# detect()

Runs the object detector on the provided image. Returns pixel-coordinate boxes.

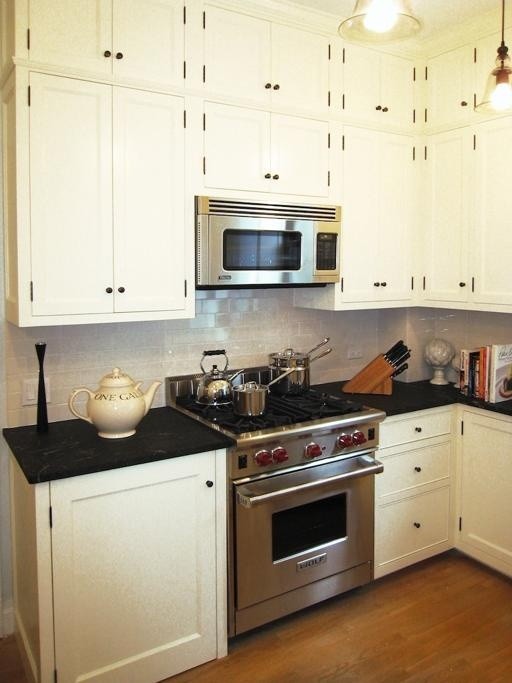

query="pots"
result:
[231,366,295,417]
[269,336,333,394]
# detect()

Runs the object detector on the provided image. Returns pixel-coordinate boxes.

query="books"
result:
[459,344,512,404]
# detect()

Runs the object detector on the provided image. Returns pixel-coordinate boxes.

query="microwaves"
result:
[195,195,341,290]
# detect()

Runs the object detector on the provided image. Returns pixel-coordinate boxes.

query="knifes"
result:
[384,340,411,378]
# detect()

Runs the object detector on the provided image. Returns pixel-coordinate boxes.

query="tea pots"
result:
[194,348,246,405]
[69,366,162,438]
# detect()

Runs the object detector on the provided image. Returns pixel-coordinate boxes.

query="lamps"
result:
[479,0,512,110]
[332,0,425,45]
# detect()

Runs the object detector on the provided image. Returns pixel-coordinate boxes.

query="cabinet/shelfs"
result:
[417,117,510,316]
[293,129,416,311]
[0,0,188,85]
[418,24,512,134]
[11,450,226,683]
[458,404,512,579]
[0,70,198,328]
[375,400,458,580]
[192,1,334,112]
[195,100,337,207]
[328,37,420,135]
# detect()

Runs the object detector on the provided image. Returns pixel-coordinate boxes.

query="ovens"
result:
[227,446,387,637]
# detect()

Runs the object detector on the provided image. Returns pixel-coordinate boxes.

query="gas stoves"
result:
[176,387,387,479]
